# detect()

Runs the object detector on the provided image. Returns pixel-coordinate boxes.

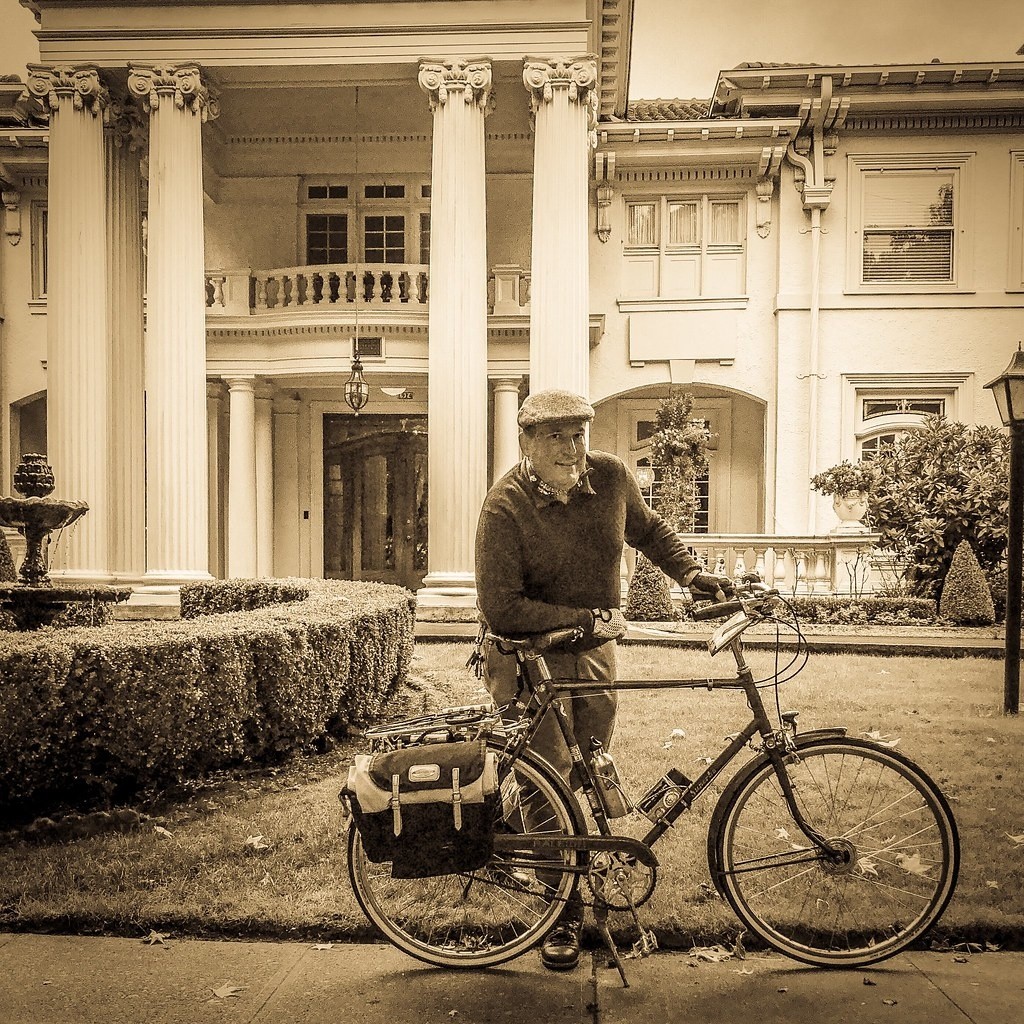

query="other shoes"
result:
[487,848,532,887]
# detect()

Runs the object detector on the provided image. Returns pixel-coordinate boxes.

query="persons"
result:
[474,387,737,970]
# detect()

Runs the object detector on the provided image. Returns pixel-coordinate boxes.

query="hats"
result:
[517,387,596,428]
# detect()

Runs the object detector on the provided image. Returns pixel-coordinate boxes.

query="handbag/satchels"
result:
[339,740,518,880]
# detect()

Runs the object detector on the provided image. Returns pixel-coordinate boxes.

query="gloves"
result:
[681,569,738,605]
[593,607,628,639]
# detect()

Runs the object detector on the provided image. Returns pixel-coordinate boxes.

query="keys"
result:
[465,649,484,680]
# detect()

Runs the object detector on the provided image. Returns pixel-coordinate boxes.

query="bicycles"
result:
[349,576,961,1023]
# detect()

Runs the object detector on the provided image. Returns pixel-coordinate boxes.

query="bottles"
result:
[588,735,633,818]
[636,768,691,824]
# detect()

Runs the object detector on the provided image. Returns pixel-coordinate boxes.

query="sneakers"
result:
[543,888,585,967]
